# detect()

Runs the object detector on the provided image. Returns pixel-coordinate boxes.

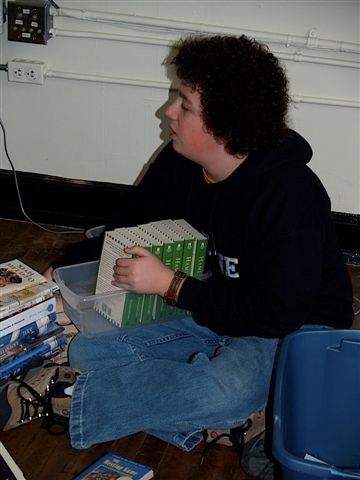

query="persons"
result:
[1,34,354,478]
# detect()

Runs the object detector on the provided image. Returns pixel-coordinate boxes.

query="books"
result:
[0,258,67,379]
[93,219,209,331]
[73,453,153,480]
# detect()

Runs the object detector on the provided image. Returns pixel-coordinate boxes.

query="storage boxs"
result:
[53,261,214,338]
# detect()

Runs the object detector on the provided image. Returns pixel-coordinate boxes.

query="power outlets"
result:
[8,59,46,84]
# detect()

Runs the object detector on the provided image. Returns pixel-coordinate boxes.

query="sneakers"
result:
[203,406,274,478]
[0,356,80,432]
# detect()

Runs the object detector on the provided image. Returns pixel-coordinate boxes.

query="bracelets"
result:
[163,270,187,307]
